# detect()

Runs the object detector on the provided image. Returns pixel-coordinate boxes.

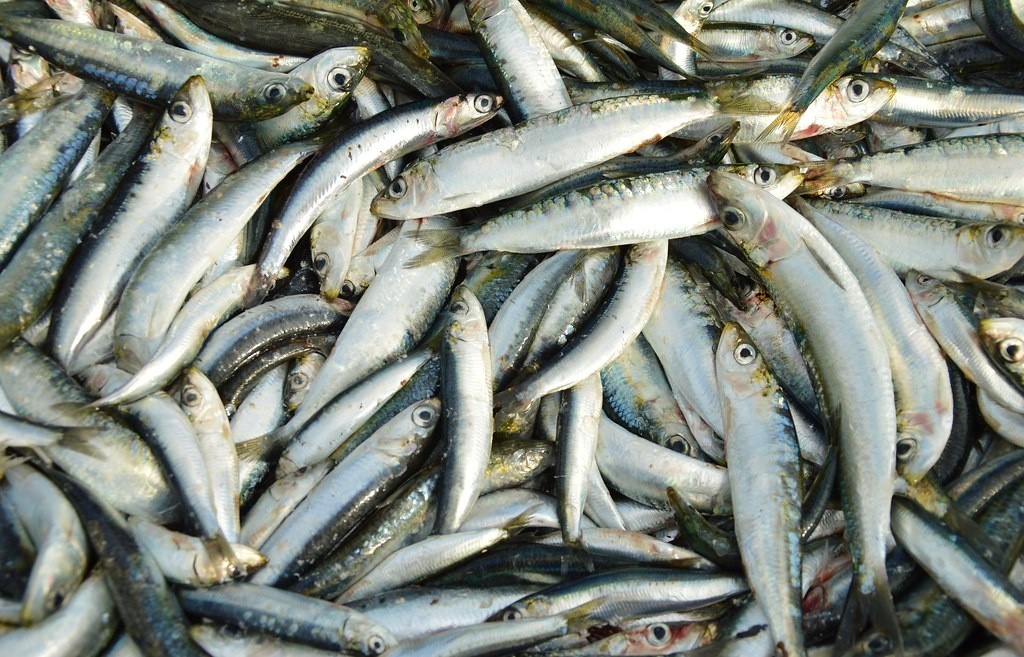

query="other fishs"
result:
[0,0,1024,657]
